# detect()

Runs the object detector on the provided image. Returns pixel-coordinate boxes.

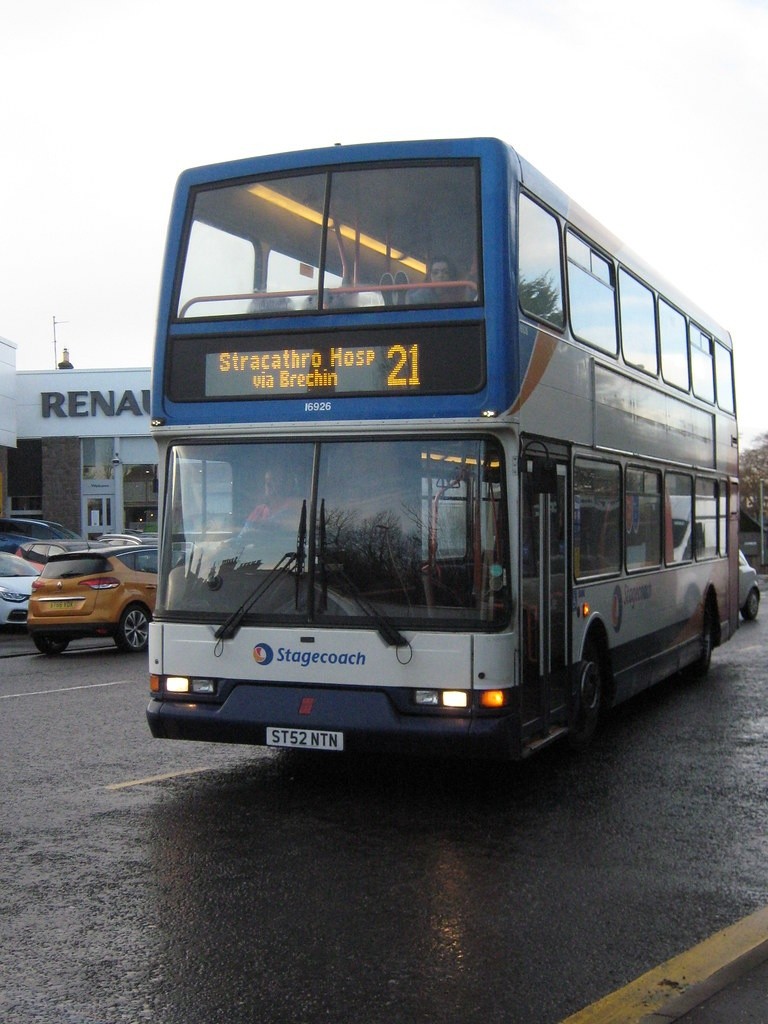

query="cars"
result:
[27,544,188,655]
[0,518,84,556]
[14,539,113,575]
[738,549,761,621]
[0,551,43,630]
[96,529,160,548]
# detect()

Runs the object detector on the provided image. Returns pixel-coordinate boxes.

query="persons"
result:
[216,462,307,569]
[379,255,466,305]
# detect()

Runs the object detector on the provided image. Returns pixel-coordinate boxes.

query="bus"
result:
[149,136,742,759]
